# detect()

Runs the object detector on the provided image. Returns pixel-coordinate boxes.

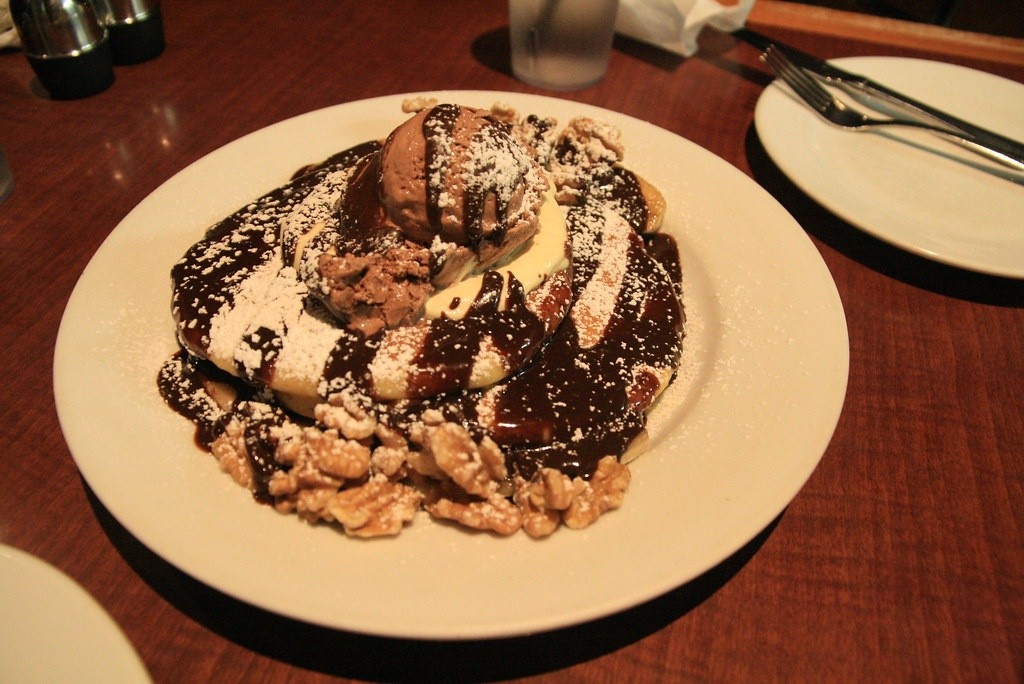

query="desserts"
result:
[154,95,687,540]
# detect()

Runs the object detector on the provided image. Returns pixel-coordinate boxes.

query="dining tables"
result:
[5,0,1021,684]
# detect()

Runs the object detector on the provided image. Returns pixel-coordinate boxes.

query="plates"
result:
[51,91,850,639]
[0,542,152,684]
[752,56,1024,279]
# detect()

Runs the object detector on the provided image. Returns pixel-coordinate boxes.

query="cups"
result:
[509,0,620,90]
[8,1,116,100]
[89,0,166,68]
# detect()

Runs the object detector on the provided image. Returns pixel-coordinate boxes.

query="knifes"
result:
[731,26,1023,160]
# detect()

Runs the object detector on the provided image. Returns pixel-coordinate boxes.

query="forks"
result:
[760,44,1024,169]
